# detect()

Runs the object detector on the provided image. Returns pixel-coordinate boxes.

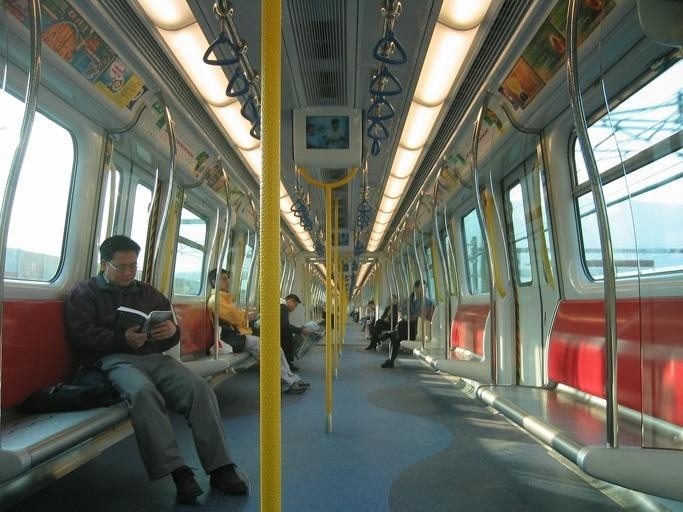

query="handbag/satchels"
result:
[416,317,430,342]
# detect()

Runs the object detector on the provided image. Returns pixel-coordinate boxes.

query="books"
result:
[114,306,171,343]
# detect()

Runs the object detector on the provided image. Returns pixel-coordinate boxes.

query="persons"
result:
[207,269,313,393]
[351,280,432,368]
[279,294,309,370]
[67,235,247,501]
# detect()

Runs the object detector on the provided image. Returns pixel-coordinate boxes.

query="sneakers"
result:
[283,364,312,397]
[169,466,202,501]
[364,333,396,370]
[210,463,247,494]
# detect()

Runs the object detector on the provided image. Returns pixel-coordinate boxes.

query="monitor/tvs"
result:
[343,262,351,297]
[293,105,363,168]
[331,229,355,250]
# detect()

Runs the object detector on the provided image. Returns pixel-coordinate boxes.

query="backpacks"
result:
[28,361,119,412]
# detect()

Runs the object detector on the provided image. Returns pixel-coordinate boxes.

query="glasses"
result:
[104,259,139,273]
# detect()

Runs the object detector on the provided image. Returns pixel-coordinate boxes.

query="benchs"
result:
[474,296,682,501]
[399,295,495,387]
[0,294,263,512]
